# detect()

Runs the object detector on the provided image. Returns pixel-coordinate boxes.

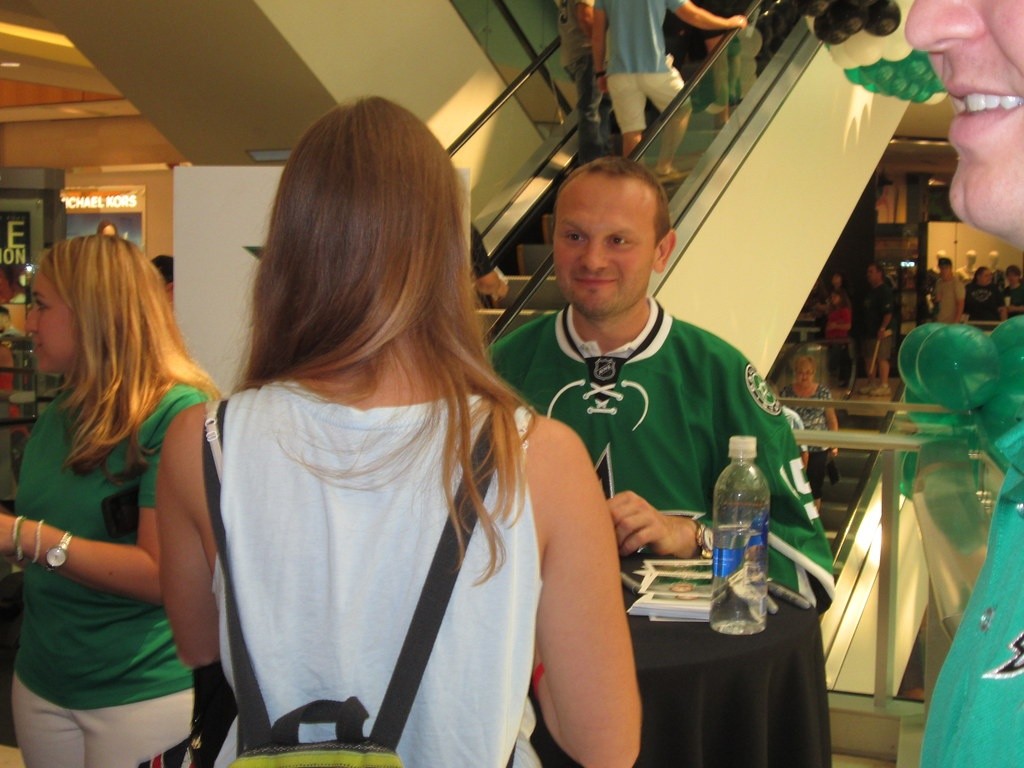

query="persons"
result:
[551,0,756,191]
[0,234,228,768]
[763,255,1024,513]
[157,95,642,768]
[905,0,1024,768]
[485,154,835,618]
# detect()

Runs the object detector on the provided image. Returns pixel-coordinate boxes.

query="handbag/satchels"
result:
[826,459,840,486]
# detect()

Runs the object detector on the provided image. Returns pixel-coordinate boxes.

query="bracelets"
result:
[12,516,27,563]
[32,520,45,564]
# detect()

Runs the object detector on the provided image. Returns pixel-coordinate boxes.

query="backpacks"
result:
[166,393,511,768]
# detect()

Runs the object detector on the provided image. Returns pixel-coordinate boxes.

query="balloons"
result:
[737,0,804,95]
[796,0,951,106]
[894,313,1024,551]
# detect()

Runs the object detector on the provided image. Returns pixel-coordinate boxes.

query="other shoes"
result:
[653,164,680,184]
[858,383,891,396]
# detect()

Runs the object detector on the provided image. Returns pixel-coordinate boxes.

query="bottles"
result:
[709,434,768,635]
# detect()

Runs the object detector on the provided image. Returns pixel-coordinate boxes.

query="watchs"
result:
[45,530,72,575]
[695,521,714,559]
